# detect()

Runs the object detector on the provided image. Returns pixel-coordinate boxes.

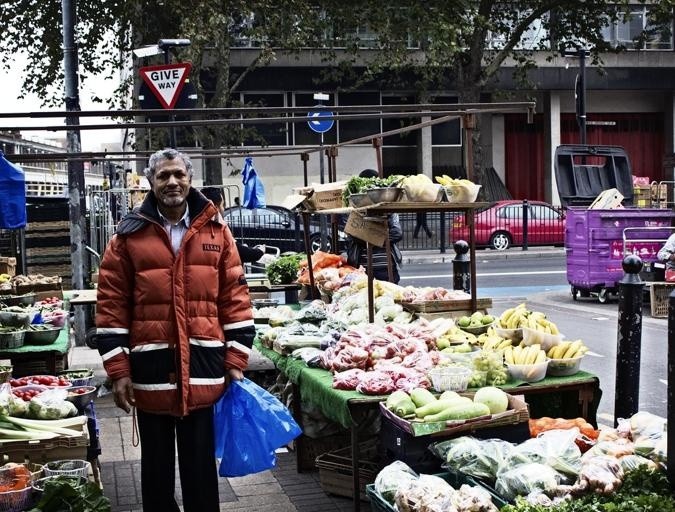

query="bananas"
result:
[441,303,590,378]
[435,173,476,201]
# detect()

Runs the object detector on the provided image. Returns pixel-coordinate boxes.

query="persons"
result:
[413,212,435,239]
[657,232,675,272]
[96,149,258,510]
[337,170,404,287]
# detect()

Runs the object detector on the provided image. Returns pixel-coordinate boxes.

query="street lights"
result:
[558,40,591,166]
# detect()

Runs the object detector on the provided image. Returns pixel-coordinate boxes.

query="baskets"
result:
[650,284,674,317]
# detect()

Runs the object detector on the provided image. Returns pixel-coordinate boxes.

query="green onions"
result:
[0,413,87,443]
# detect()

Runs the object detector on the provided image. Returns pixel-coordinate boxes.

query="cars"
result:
[222,206,348,254]
[449,198,567,250]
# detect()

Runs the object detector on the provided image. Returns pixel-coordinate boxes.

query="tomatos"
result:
[9,375,89,401]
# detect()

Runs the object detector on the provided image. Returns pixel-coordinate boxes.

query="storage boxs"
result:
[380,384,532,472]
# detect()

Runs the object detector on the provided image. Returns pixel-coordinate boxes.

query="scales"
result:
[245,273,271,290]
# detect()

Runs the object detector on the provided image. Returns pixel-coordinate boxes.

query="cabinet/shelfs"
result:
[290,196,492,332]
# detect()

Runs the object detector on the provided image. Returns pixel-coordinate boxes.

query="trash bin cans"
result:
[554,145,675,303]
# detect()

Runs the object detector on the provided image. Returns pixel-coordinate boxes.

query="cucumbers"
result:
[386,388,507,423]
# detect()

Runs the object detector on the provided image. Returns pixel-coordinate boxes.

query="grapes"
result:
[468,352,512,387]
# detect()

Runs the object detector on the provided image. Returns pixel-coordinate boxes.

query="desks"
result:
[251,337,602,510]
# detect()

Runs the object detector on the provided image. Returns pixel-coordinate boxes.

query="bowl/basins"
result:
[0,457,91,510]
[10,369,98,410]
[0,290,61,348]
[345,184,482,209]
[429,312,587,391]
